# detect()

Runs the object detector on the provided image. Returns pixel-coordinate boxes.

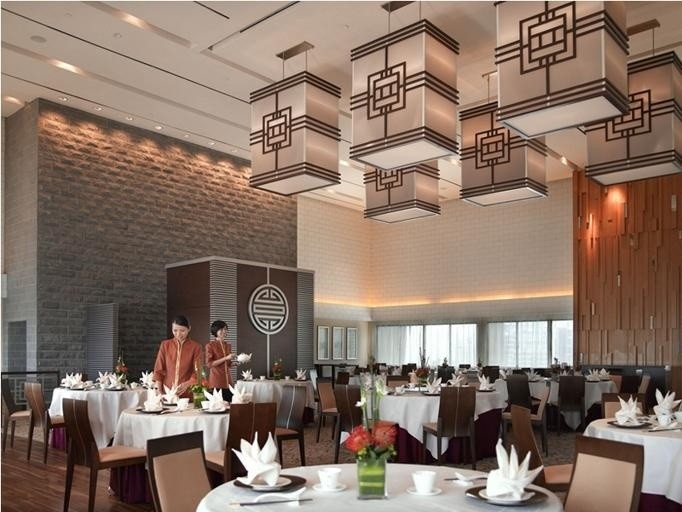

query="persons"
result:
[153,315,203,403]
[205,320,238,400]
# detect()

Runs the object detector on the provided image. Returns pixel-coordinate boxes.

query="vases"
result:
[419,378,428,389]
[356,459,387,499]
[275,373,282,380]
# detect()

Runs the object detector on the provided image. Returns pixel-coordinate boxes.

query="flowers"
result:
[416,347,436,378]
[345,355,398,460]
[273,358,283,373]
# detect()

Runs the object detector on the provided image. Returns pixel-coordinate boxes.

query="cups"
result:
[412,471,437,493]
[395,386,406,394]
[86,381,92,385]
[130,382,140,390]
[318,468,342,488]
[179,398,189,410]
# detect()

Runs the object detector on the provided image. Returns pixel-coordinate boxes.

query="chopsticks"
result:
[230,499,314,507]
[444,477,488,481]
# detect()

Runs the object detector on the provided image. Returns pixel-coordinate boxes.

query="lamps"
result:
[582,23,677,187]
[347,1,459,172]
[458,73,549,208]
[247,46,341,197]
[361,160,441,224]
[495,2,633,140]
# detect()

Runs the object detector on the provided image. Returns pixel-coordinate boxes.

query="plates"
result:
[141,409,163,414]
[313,484,346,492]
[202,408,230,414]
[234,475,306,493]
[607,420,652,428]
[422,392,440,396]
[407,487,442,496]
[477,388,496,392]
[465,486,550,506]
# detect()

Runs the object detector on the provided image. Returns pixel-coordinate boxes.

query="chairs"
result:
[510,404,574,492]
[333,385,364,463]
[501,380,552,457]
[512,364,571,379]
[563,432,644,512]
[277,385,307,467]
[205,401,277,470]
[320,361,417,393]
[601,391,647,422]
[102,396,103,398]
[60,397,147,492]
[145,430,213,492]
[316,376,341,442]
[22,380,66,463]
[506,374,541,410]
[554,376,586,434]
[2,377,37,454]
[422,386,477,468]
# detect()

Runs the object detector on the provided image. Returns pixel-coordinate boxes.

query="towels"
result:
[448,373,463,387]
[560,370,569,376]
[140,371,153,384]
[528,371,537,381]
[654,388,681,427]
[96,371,110,385]
[615,395,642,425]
[65,372,73,386]
[487,437,544,500]
[229,384,251,403]
[424,377,442,394]
[477,374,495,390]
[202,388,226,411]
[587,368,600,382]
[295,369,307,380]
[241,368,252,380]
[109,373,123,389]
[164,383,178,404]
[144,387,162,411]
[600,368,609,379]
[70,373,83,387]
[231,431,281,487]
